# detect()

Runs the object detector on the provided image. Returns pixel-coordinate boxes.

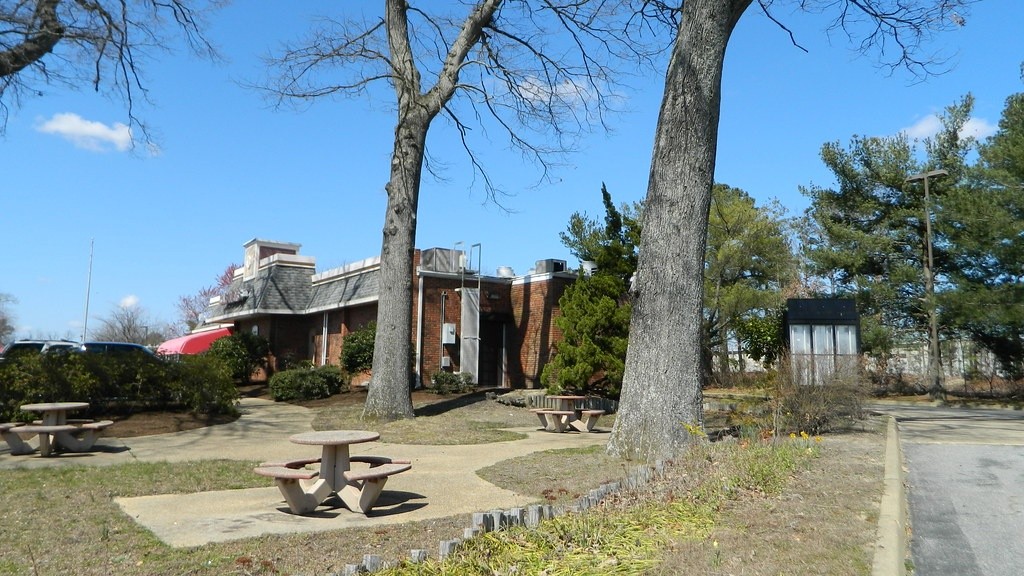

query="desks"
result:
[289,430,380,514]
[545,394,586,433]
[20,401,90,454]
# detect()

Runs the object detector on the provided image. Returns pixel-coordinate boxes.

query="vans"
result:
[0,339,81,383]
[40,341,187,407]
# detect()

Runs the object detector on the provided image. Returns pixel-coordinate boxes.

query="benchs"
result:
[529,407,605,433]
[253,453,412,514]
[0,418,114,456]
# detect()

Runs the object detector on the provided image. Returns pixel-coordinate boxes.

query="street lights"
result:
[906,167,952,398]
